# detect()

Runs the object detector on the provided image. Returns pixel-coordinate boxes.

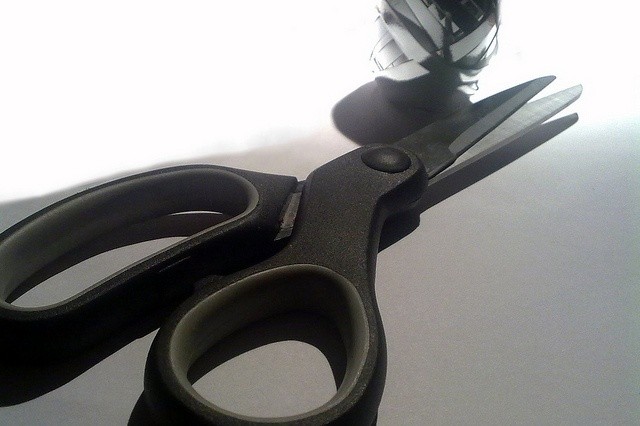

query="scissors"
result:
[1,75,582,426]
